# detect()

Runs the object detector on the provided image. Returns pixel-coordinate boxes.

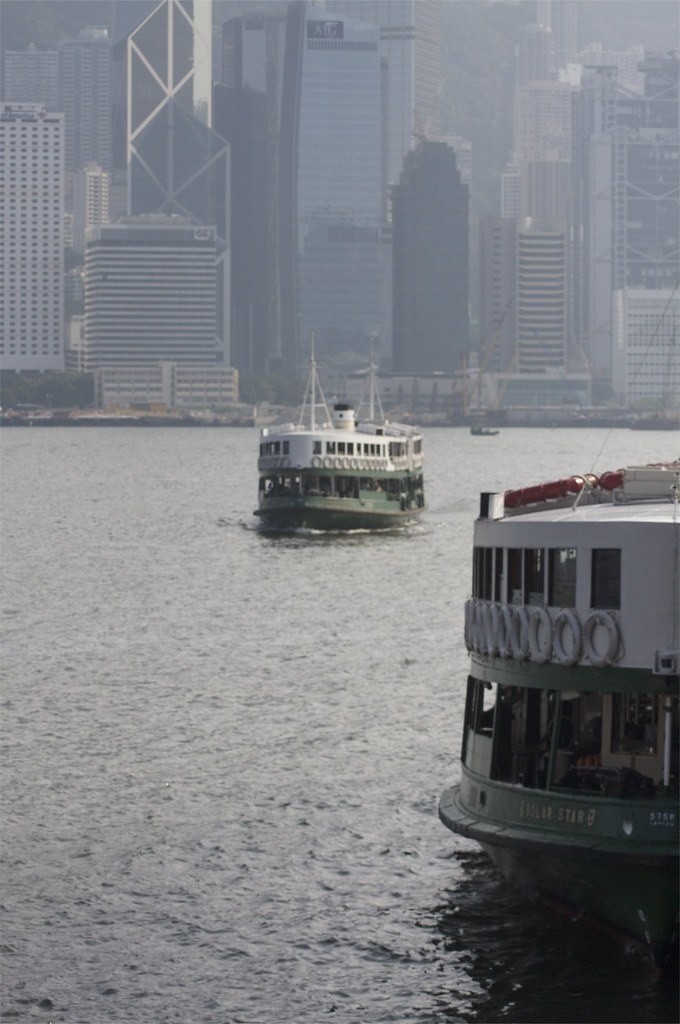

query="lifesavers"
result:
[582,612,620,668]
[257,456,291,469]
[343,457,389,471]
[528,607,553,665]
[553,608,582,668]
[333,457,342,469]
[311,456,322,468]
[510,606,529,661]
[323,457,333,469]
[463,599,509,657]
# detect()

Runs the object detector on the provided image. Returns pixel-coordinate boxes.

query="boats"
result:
[252,330,429,535]
[434,453,680,964]
